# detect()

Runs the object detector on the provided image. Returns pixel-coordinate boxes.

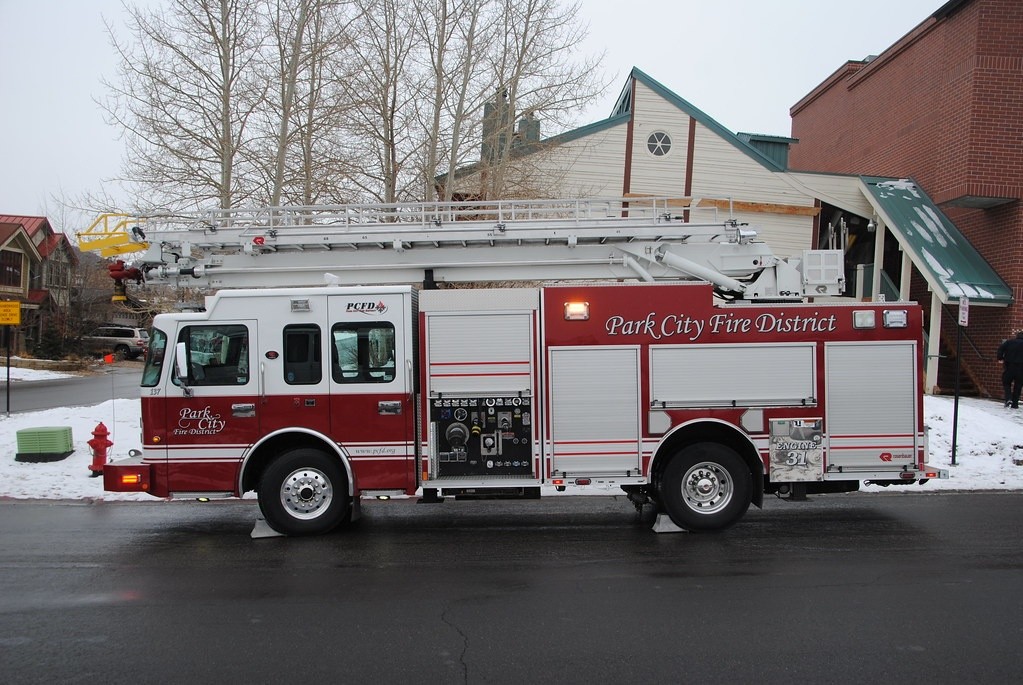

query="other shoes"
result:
[1004,398,1013,407]
[1011,406,1019,408]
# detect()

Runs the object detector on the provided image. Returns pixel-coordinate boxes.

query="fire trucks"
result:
[74,193,957,541]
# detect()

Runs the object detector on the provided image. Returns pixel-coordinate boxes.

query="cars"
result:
[143,339,166,365]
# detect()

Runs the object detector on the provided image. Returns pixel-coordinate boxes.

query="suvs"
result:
[78,324,151,362]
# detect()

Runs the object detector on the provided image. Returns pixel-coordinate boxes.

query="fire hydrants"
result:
[86,421,115,477]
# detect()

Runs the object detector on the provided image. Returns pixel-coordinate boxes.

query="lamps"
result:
[868,218,878,232]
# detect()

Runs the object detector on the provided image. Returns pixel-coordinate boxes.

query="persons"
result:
[997,331,1023,409]
